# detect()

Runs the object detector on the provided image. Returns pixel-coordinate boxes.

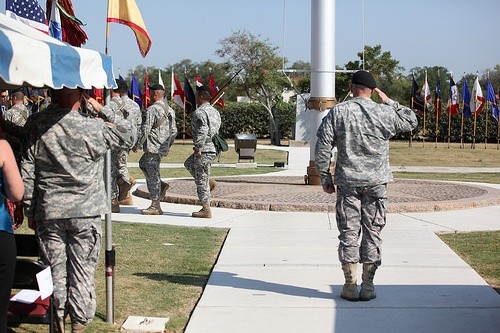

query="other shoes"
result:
[71,323,83,333]
[52,318,65,333]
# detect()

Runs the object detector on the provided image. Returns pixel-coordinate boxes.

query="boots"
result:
[140,200,162,215]
[192,201,212,218]
[130,177,136,188]
[160,181,169,198]
[359,264,376,301]
[118,190,132,205]
[341,262,360,301]
[208,179,217,191]
[111,199,120,213]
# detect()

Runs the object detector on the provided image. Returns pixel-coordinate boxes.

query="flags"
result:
[5,0,224,113]
[410,71,500,121]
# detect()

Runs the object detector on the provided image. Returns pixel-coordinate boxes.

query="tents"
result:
[0,13,118,89]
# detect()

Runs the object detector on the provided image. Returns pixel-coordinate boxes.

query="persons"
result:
[0,84,220,333]
[316,71,419,301]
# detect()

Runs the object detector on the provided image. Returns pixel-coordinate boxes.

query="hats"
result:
[150,85,164,90]
[352,70,377,90]
[199,86,213,95]
[113,78,121,87]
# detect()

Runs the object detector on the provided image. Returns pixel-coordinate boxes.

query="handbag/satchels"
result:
[212,133,228,155]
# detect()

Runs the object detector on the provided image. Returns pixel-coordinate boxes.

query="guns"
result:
[209,69,242,107]
